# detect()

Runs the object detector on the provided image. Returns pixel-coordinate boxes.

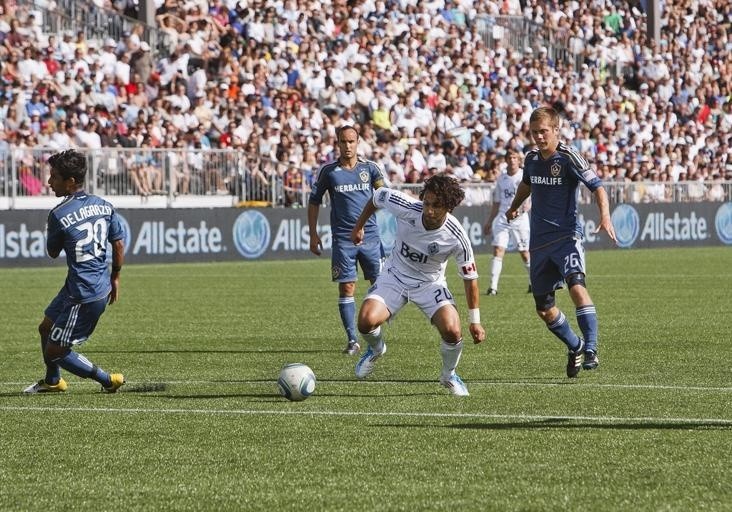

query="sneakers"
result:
[486,288,496,294]
[23,377,68,393]
[343,339,361,355]
[583,348,599,370]
[567,337,586,377]
[355,340,387,379]
[440,374,469,395]
[100,373,124,393]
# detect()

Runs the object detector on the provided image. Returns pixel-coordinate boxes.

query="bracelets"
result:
[468,308,481,323]
[112,264,121,271]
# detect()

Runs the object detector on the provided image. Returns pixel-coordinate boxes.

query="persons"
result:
[351,175,486,395]
[308,126,389,357]
[484,149,533,295]
[24,149,126,393]
[1,1,732,210]
[505,107,618,378]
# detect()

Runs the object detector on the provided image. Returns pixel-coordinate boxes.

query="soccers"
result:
[278,363,316,401]
